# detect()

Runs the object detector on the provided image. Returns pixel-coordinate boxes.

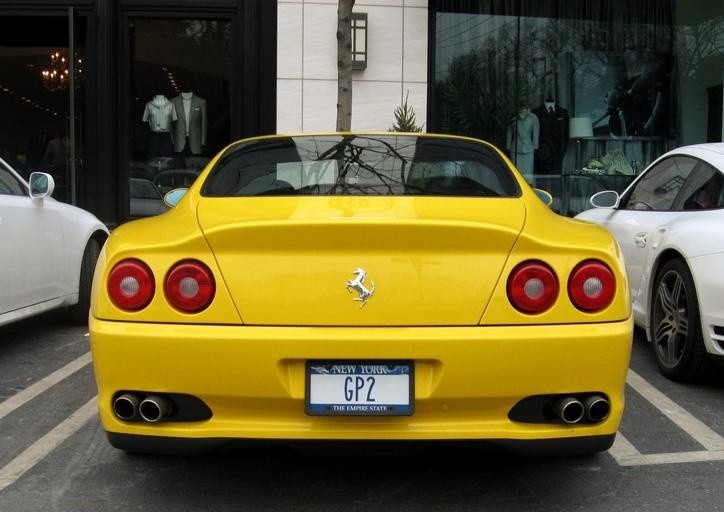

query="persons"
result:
[141,94,178,155]
[504,103,541,178]
[167,91,209,158]
[530,97,569,176]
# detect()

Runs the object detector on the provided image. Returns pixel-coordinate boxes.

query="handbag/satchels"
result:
[608,150,635,176]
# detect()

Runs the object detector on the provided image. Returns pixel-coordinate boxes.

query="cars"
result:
[571,140,724,383]
[0,154,113,333]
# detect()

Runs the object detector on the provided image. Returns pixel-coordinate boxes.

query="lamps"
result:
[40,50,87,93]
[569,117,593,175]
[351,12,368,70]
